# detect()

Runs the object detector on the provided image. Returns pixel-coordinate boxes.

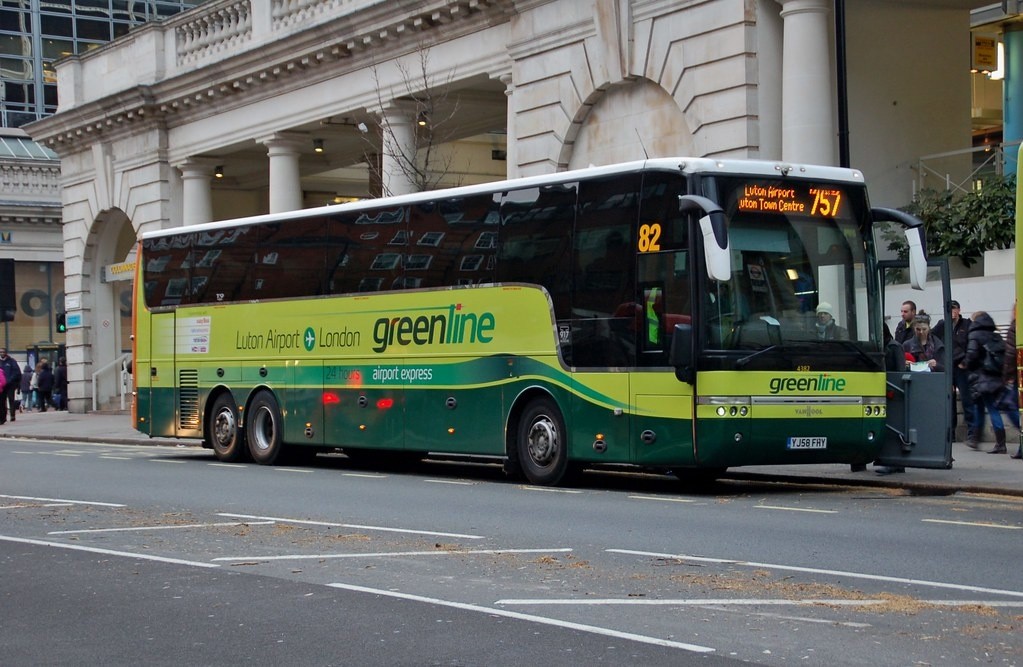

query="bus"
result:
[131,156,925,486]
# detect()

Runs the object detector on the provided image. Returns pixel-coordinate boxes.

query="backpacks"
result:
[12,371,21,389]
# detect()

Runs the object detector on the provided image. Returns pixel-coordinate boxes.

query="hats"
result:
[815,301,833,318]
[950,300,960,309]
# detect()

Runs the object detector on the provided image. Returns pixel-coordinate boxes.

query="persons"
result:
[0,343,67,425]
[931,300,980,448]
[1003,298,1023,459]
[875,321,905,475]
[894,302,919,345]
[811,302,850,342]
[957,310,1021,454]
[901,310,946,372]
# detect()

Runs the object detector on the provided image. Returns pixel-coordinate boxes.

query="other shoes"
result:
[11,414,15,421]
[1011,448,1023,459]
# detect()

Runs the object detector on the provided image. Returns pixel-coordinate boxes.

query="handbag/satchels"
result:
[980,334,1006,375]
[51,393,61,407]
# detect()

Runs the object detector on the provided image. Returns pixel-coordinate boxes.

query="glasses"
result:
[912,314,930,320]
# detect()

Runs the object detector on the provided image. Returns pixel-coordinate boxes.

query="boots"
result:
[962,427,983,448]
[986,426,1007,454]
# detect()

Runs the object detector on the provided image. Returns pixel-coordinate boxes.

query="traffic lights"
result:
[55,312,66,333]
